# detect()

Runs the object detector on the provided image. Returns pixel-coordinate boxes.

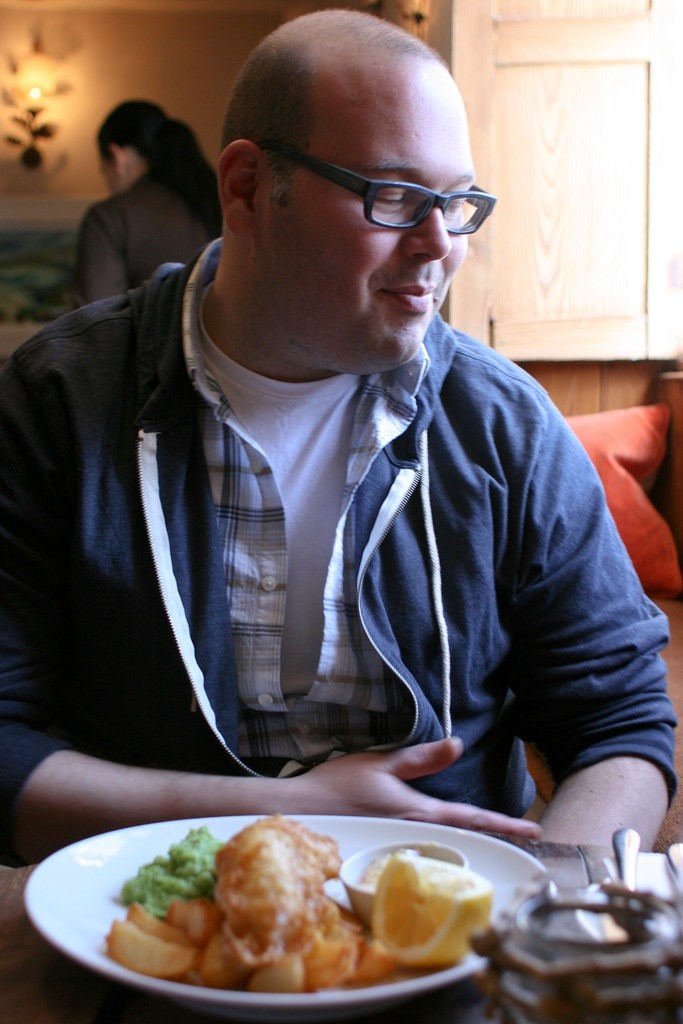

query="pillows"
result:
[557,404,683,597]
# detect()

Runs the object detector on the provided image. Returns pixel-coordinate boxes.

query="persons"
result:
[74,99,224,310]
[0,8,677,861]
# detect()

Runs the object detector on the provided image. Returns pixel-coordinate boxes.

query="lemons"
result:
[370,851,495,968]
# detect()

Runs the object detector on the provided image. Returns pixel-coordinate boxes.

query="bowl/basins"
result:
[339,839,470,926]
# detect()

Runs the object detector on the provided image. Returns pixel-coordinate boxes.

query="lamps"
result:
[0,43,72,164]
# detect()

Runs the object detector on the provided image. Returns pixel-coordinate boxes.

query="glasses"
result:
[263,140,497,235]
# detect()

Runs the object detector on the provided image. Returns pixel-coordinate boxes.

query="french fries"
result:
[108,896,396,994]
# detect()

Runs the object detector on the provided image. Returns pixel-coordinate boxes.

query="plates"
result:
[23,813,552,1023]
